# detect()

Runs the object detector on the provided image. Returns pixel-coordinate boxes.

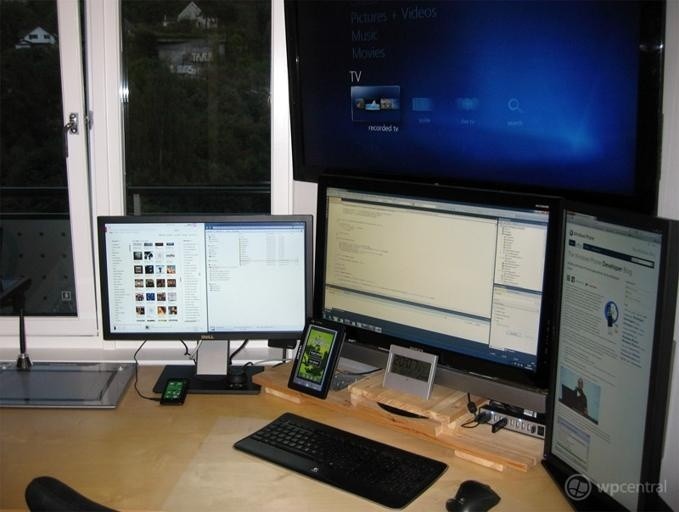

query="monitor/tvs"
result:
[541,192,679,511]
[284,0,666,217]
[98,215,313,395]
[314,174,563,414]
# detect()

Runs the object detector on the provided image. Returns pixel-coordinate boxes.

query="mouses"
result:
[446,480,500,512]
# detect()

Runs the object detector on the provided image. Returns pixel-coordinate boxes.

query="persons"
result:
[570,377,588,415]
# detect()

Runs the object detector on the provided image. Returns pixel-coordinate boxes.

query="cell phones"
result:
[160,378,189,405]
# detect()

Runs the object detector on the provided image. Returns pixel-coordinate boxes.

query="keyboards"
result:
[231,412,447,509]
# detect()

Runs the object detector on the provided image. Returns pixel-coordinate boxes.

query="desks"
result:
[2,363,580,511]
